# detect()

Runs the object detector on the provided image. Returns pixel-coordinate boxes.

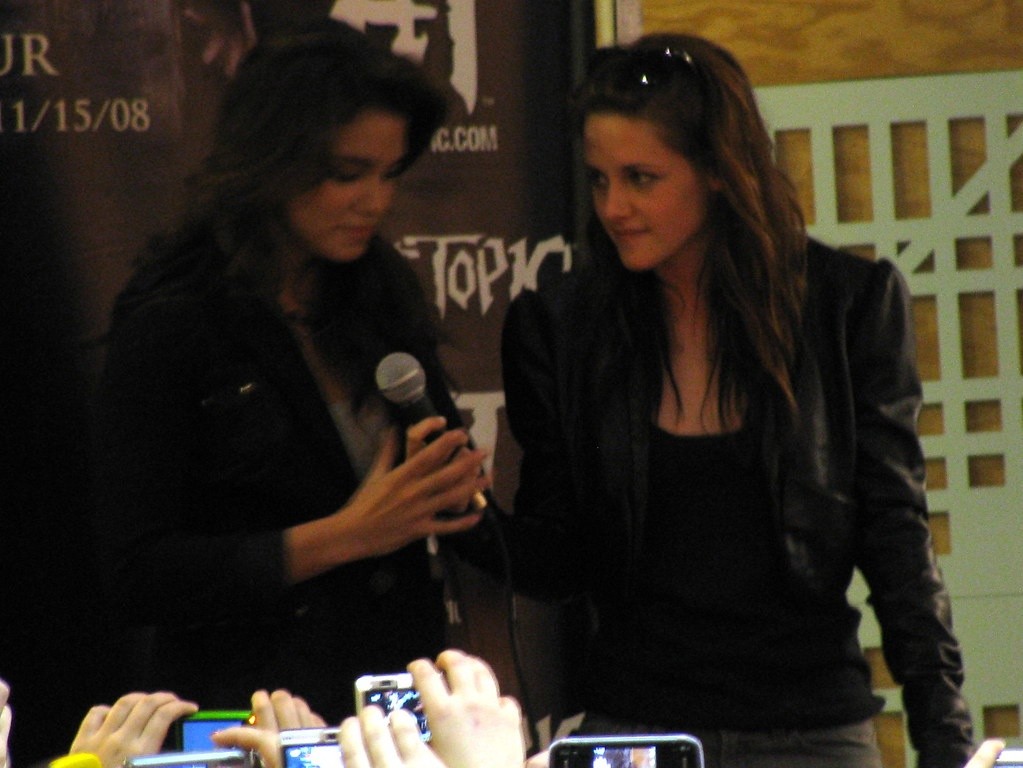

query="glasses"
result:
[585,42,708,93]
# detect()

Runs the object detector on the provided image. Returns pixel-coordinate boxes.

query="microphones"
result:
[377,353,490,515]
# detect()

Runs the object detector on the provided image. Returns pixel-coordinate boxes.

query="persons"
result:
[55,648,547,768]
[499,33,977,768]
[84,13,492,728]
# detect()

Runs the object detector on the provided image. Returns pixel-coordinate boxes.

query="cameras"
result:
[280,729,345,768]
[124,710,258,768]
[355,671,448,743]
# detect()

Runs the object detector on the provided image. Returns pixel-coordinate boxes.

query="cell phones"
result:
[546,734,705,768]
